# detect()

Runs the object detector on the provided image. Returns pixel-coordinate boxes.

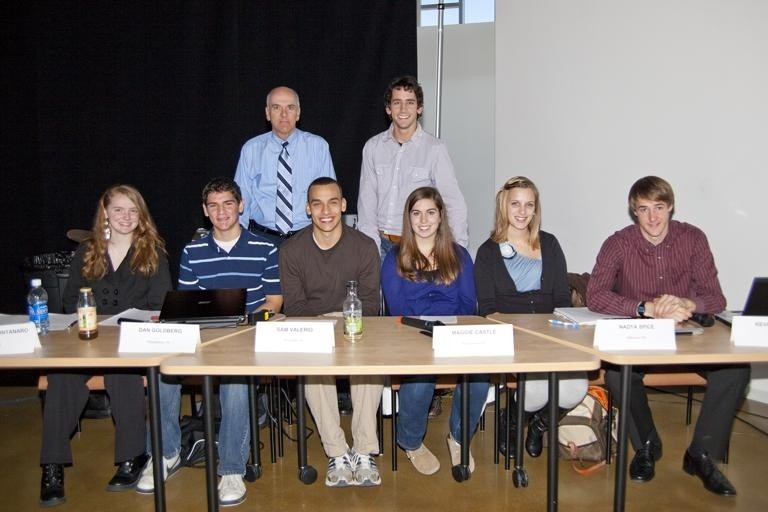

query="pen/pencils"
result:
[676,332,693,335]
[67,320,78,330]
[547,319,573,326]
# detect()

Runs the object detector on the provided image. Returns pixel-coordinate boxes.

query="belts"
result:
[250,221,295,240]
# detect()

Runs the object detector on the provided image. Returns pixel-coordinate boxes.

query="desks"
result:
[0,308,285,512]
[485,312,768,512]
[160,312,601,511]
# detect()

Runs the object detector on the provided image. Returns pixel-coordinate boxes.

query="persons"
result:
[234,87,337,246]
[382,188,490,474]
[357,76,468,256]
[278,178,382,487]
[586,177,750,496]
[35,185,173,506]
[137,179,281,506]
[473,176,589,459]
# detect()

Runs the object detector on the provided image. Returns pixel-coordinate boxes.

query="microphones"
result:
[689,313,715,327]
[401,316,445,333]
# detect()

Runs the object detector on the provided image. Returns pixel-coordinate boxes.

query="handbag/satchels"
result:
[180,415,221,467]
[558,385,619,462]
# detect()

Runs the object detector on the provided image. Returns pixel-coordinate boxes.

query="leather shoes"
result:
[40,464,65,507]
[339,395,352,416]
[630,432,662,483]
[107,450,150,492]
[684,449,736,497]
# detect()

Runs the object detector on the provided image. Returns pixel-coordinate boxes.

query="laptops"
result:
[151,287,247,323]
[714,277,768,328]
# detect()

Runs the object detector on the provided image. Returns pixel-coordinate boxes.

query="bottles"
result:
[28,278,50,335]
[344,279,363,342]
[77,287,99,340]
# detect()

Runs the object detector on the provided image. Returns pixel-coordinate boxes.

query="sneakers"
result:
[217,474,248,507]
[427,392,441,419]
[405,442,441,476]
[351,445,381,486]
[325,442,353,487]
[446,432,475,474]
[136,454,181,495]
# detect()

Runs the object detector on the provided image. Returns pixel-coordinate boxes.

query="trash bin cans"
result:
[23,250,78,314]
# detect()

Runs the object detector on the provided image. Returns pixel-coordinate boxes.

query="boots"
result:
[499,390,536,459]
[526,401,573,457]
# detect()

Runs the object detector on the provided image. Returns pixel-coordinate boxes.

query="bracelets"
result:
[638,301,647,316]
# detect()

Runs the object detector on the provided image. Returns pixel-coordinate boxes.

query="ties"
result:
[276,140,293,237]
